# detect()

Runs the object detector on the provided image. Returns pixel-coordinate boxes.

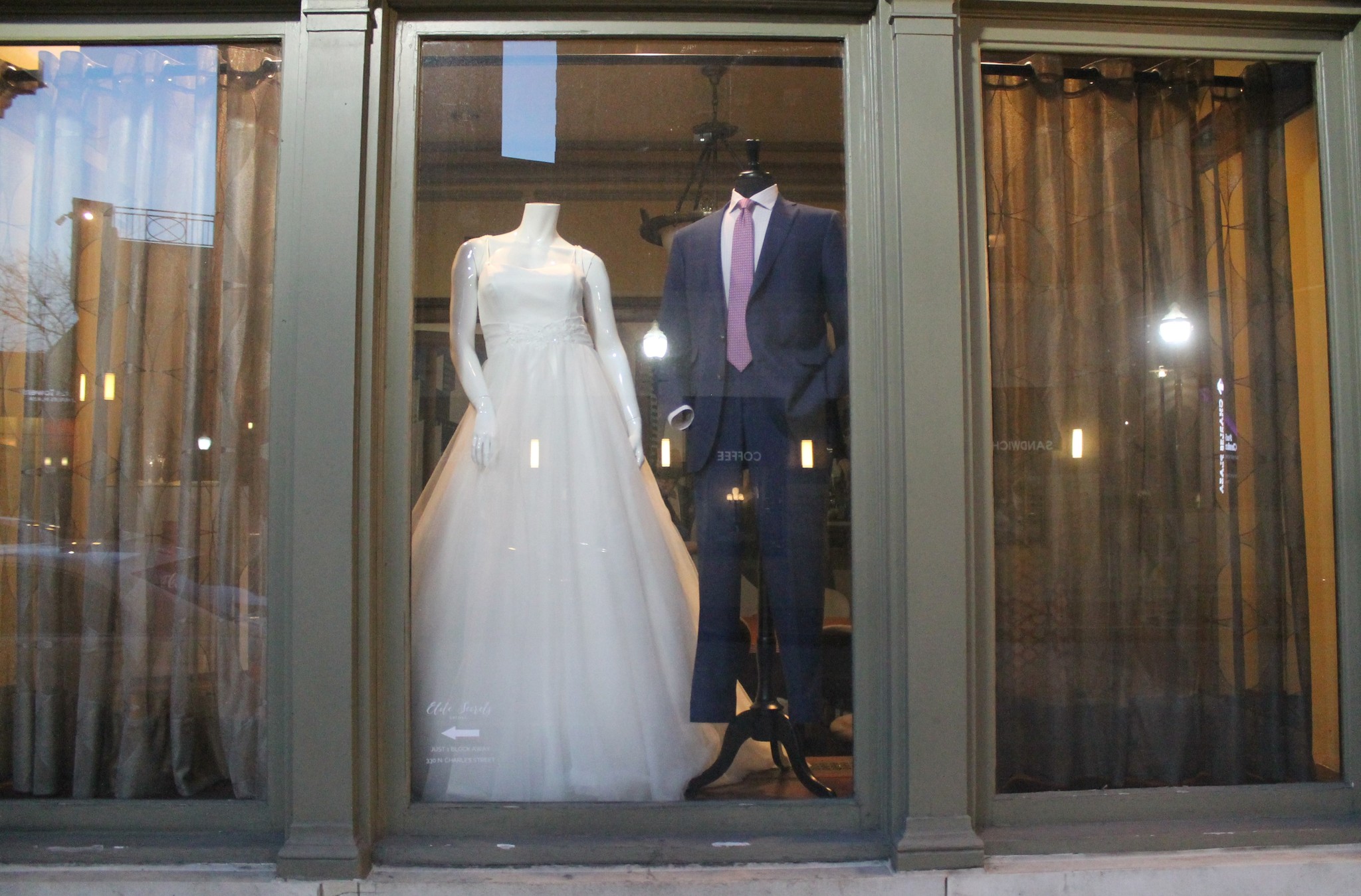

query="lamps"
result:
[639,65,748,251]
[641,320,668,357]
[1159,302,1193,343]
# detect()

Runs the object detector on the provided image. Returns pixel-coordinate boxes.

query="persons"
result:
[410,203,787,802]
[653,168,848,728]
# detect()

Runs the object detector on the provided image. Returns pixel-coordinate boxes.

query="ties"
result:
[727,199,754,372]
